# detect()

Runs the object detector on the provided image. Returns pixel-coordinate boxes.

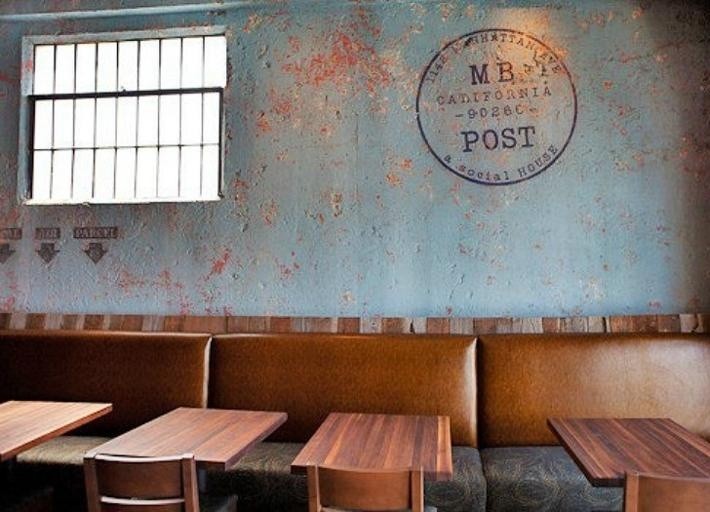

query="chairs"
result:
[306,461,438,512]
[622,468,708,511]
[81,454,200,511]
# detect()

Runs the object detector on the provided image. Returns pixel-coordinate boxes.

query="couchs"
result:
[479,330,709,512]
[209,331,488,512]
[1,328,213,512]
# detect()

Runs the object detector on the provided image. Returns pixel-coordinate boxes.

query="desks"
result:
[86,407,289,472]
[545,417,709,489]
[291,411,455,482]
[1,399,114,462]
